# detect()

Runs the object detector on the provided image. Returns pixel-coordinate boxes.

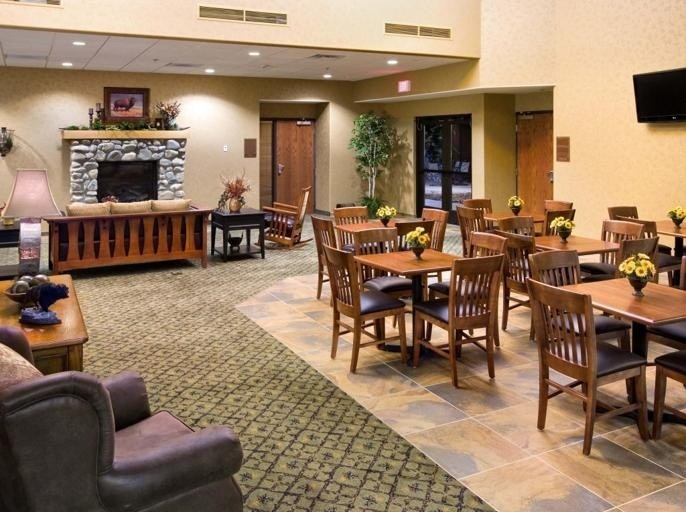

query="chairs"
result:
[254,185,313,250]
[314,196,685,303]
[525,278,650,454]
[322,243,408,374]
[426,230,508,356]
[352,227,419,335]
[500,231,541,341]
[412,254,507,389]
[1,325,245,512]
[529,250,634,410]
[651,348,686,443]
[646,255,686,369]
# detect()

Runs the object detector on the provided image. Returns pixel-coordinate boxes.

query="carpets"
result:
[0,228,501,512]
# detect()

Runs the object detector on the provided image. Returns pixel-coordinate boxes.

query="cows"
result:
[21,282,69,312]
[112,96,137,112]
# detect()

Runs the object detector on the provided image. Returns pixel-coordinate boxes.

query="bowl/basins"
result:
[2,283,68,312]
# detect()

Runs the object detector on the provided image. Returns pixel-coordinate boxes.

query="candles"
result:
[89,108,93,114]
[96,103,101,111]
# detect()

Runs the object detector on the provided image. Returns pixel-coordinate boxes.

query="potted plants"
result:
[214,170,252,214]
[152,100,182,129]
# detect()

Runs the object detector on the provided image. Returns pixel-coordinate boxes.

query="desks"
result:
[210,207,269,262]
[0,273,89,375]
[351,248,464,356]
[552,277,685,443]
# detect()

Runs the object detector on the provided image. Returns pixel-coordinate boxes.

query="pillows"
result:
[150,200,192,212]
[111,200,151,214]
[66,201,114,216]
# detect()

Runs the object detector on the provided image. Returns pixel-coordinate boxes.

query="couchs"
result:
[43,205,213,275]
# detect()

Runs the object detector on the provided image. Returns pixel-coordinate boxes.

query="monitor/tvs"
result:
[633,68,686,121]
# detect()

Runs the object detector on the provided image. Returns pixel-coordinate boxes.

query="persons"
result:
[425,143,442,183]
[437,136,460,184]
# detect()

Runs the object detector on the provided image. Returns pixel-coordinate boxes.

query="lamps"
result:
[1,169,62,276]
[0,127,15,156]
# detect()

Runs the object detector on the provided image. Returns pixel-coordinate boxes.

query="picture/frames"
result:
[103,87,151,124]
